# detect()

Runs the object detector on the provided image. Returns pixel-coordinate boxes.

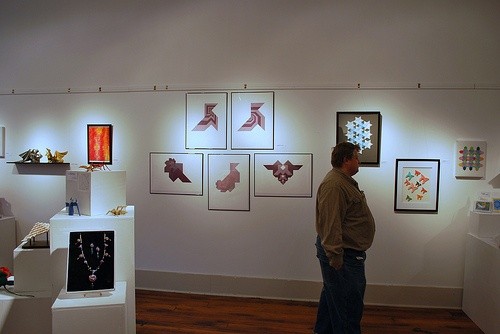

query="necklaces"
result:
[77,233,110,283]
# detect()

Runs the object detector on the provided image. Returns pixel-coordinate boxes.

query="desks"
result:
[462,233,500,334]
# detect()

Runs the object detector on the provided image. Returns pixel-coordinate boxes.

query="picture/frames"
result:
[335,111,381,166]
[231,91,274,150]
[87,124,112,164]
[394,159,440,212]
[150,152,203,196]
[185,92,227,150]
[254,153,313,198]
[208,154,250,211]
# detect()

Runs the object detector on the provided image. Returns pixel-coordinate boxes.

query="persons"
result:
[314,142,376,334]
